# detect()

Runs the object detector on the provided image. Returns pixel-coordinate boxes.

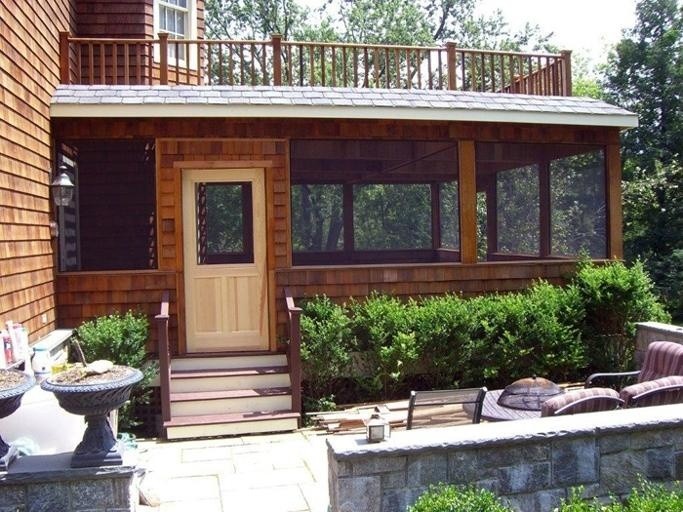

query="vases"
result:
[0,369,37,471]
[41,362,146,466]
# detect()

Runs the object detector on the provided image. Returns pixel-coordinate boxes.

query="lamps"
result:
[41,165,73,208]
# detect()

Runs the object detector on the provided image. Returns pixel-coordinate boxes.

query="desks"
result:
[465,388,542,420]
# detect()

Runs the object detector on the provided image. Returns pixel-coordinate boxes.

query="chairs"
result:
[541,387,624,415]
[406,386,487,428]
[620,375,682,407]
[584,341,683,385]
